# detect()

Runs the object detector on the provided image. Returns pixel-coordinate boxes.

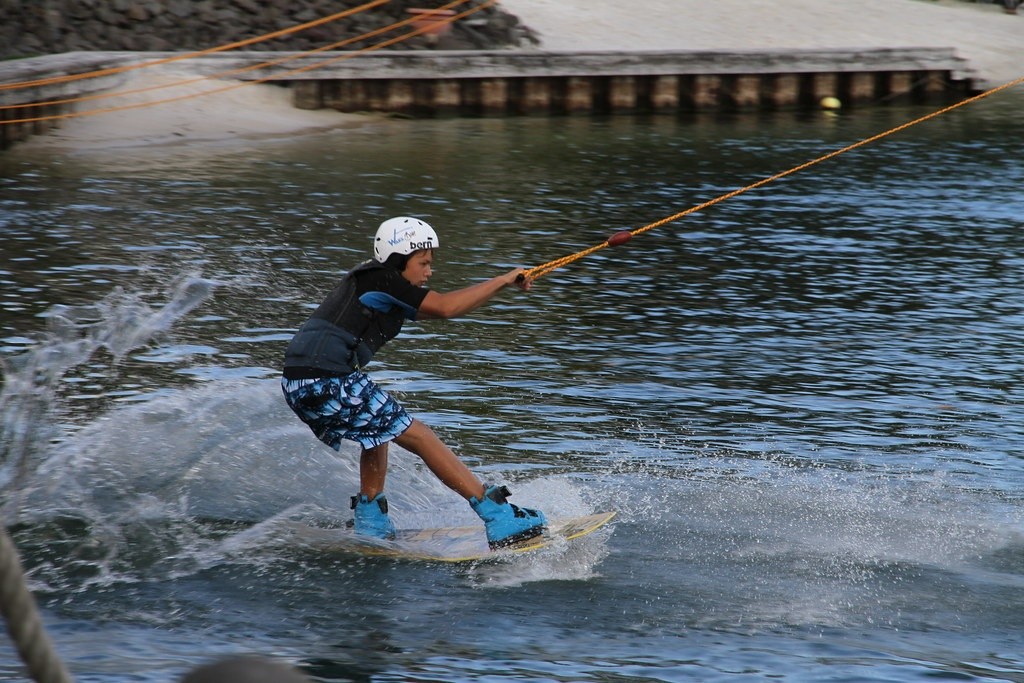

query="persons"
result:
[280,216,548,550]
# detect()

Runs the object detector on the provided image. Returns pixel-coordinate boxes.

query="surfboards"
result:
[324,509,617,563]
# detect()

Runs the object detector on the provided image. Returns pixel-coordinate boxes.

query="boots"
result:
[350,494,395,542]
[469,481,545,547]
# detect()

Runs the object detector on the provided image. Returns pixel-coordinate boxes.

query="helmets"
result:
[373,217,439,263]
